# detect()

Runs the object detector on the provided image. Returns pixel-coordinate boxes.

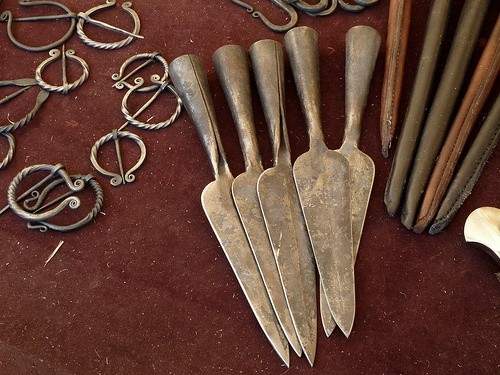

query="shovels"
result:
[215,44,303,360]
[244,39,316,367]
[318,25,381,337]
[170,53,289,366]
[281,25,358,338]
